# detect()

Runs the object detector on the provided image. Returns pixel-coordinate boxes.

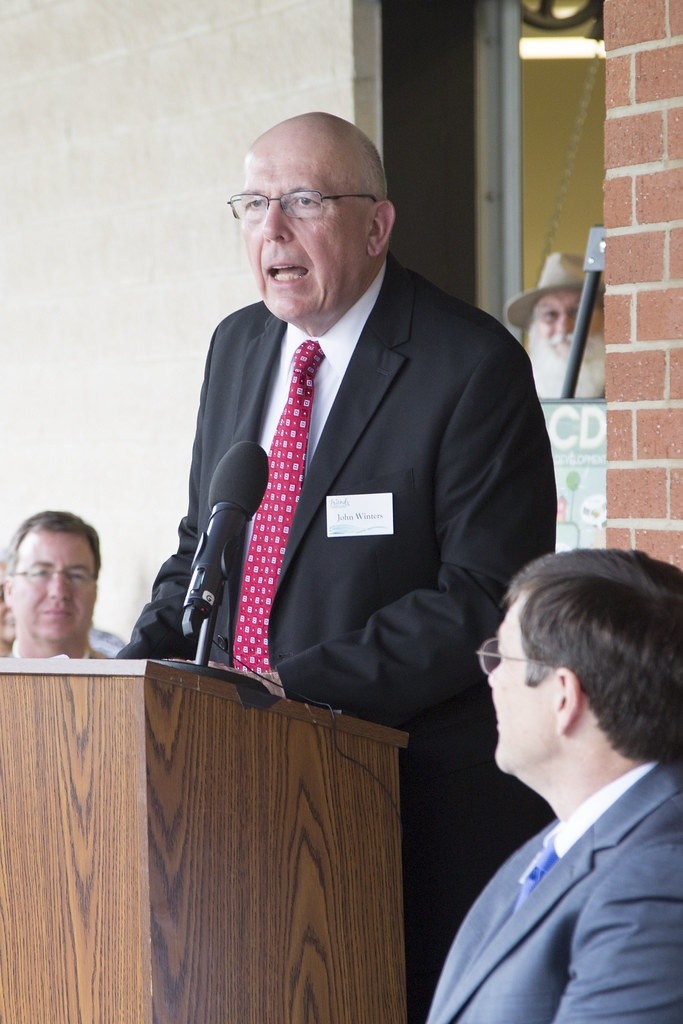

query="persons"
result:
[505,251,606,396]
[424,551,682,1024]
[115,110,558,1023]
[0,510,126,661]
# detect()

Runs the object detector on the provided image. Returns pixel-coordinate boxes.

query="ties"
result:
[513,836,561,914]
[232,339,325,674]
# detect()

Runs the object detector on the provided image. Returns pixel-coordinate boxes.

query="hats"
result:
[506,252,606,327]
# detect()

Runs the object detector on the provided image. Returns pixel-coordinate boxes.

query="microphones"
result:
[180,441,268,637]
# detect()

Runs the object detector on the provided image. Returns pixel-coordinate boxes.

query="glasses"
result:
[12,565,96,590]
[227,191,377,222]
[475,637,586,694]
